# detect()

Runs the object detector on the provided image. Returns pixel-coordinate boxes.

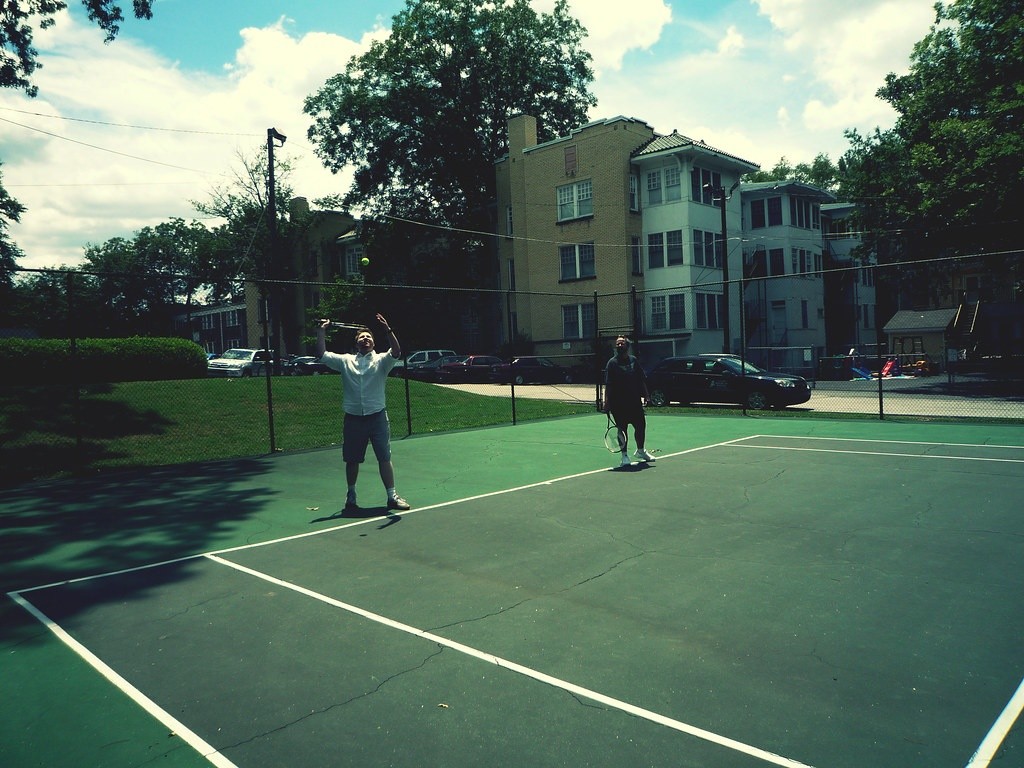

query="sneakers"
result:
[387,494,411,510]
[346,492,357,508]
[633,448,656,462]
[622,454,631,465]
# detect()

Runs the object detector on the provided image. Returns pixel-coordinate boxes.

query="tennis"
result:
[361,257,370,267]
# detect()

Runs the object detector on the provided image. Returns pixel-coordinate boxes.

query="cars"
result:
[643,354,811,410]
[207,348,333,377]
[405,351,576,384]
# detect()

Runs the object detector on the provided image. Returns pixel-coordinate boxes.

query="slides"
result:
[850,359,895,380]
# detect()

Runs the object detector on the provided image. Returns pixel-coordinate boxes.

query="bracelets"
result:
[385,327,394,334]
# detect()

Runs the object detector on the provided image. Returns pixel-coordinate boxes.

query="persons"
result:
[316,311,411,510]
[604,334,655,468]
[946,344,961,384]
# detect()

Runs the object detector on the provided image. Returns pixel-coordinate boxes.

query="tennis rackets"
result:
[313,318,366,330]
[604,410,628,453]
